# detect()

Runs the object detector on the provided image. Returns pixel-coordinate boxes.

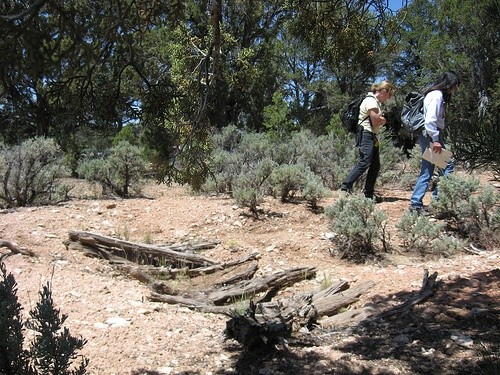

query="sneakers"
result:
[366,193,383,203]
[409,205,427,214]
[341,187,353,196]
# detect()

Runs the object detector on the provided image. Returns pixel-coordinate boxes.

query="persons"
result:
[408,69,461,218]
[339,79,394,202]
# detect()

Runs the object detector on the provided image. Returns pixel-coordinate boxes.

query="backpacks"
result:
[339,93,381,133]
[399,91,427,134]
